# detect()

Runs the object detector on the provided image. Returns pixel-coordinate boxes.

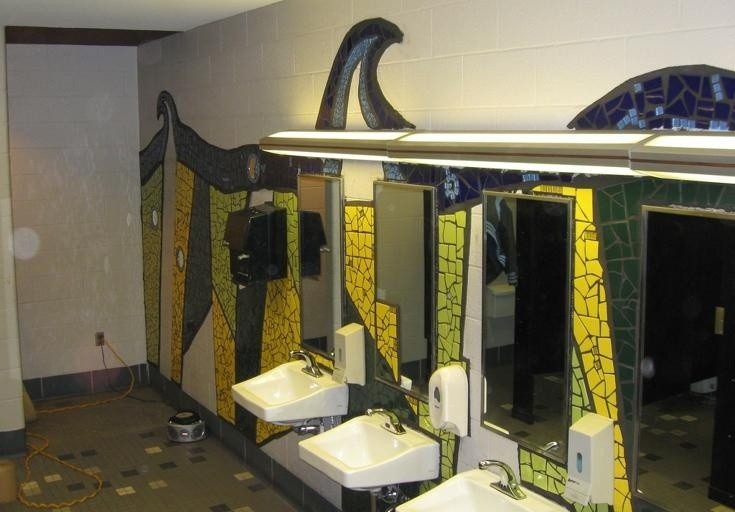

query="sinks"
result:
[395,468,571,512]
[230,359,350,426]
[296,412,441,492]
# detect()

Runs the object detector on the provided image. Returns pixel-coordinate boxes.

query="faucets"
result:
[330,346,335,357]
[478,459,527,500]
[290,349,321,378]
[541,440,560,453]
[365,407,406,435]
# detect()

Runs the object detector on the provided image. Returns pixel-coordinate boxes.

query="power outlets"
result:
[95,332,104,346]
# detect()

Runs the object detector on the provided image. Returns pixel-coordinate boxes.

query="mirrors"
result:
[630,204,735,512]
[297,175,343,362]
[372,181,436,406]
[480,191,573,470]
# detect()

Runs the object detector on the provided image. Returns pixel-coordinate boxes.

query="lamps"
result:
[259,129,411,164]
[629,132,735,185]
[388,130,654,177]
